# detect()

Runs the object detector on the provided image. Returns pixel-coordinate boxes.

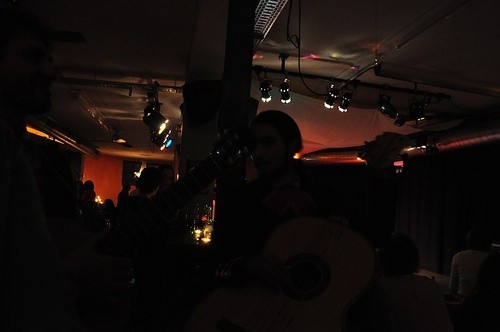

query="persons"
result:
[0,111,500,332]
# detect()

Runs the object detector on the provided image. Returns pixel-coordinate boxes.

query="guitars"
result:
[40,131,254,332]
[184,132,405,331]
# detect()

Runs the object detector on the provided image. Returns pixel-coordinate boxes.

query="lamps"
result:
[338,93,352,113]
[408,103,426,120]
[143,104,173,150]
[323,89,339,109]
[260,80,272,103]
[278,83,292,104]
[376,94,405,126]
